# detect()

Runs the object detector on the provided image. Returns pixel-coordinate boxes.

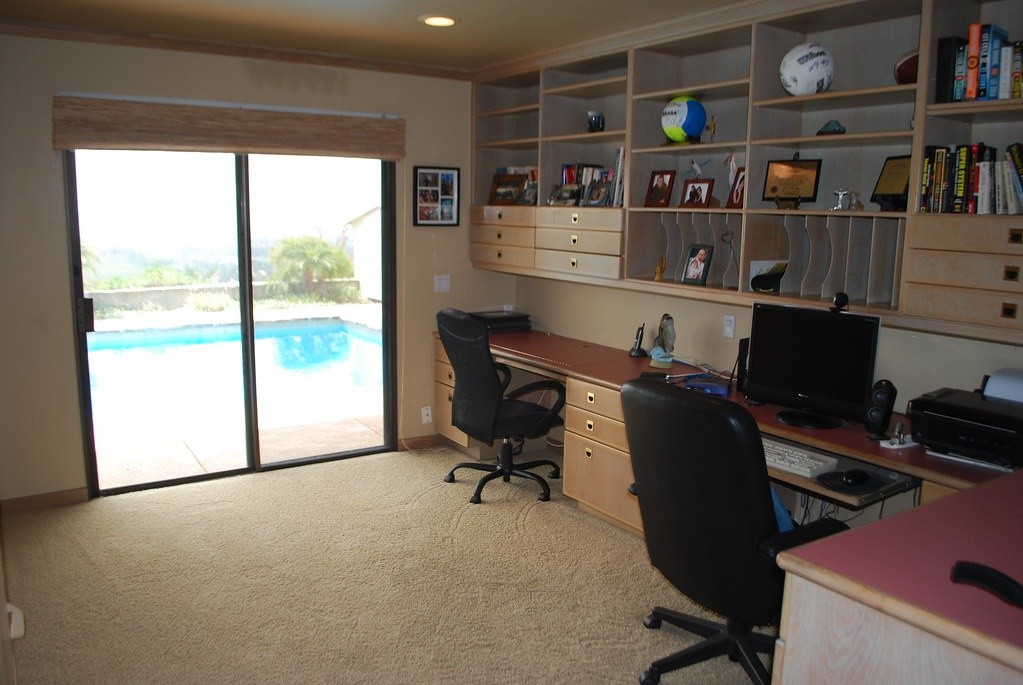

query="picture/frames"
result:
[547,183,580,208]
[487,174,528,206]
[644,169,676,207]
[583,182,610,206]
[680,177,715,208]
[725,167,745,208]
[412,165,460,226]
[681,242,713,285]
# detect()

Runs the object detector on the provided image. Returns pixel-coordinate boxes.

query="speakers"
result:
[866,378,897,441]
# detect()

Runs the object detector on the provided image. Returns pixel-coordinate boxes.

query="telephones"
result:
[628,323,648,357]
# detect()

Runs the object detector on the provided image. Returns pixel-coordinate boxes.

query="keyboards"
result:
[761,436,838,478]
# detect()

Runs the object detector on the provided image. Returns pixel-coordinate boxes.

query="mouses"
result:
[842,469,870,485]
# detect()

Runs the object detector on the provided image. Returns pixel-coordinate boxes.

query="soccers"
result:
[779,43,835,97]
[661,97,708,145]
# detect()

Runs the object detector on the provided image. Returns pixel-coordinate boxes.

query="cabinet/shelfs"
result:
[562,375,644,537]
[468,0,1023,347]
[434,340,549,458]
[769,471,1023,685]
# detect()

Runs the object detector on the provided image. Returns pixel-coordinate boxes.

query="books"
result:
[920,143,1023,215]
[937,23,1023,103]
[562,146,625,208]
[497,165,537,206]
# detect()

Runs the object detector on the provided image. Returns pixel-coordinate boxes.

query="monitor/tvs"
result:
[744,303,880,430]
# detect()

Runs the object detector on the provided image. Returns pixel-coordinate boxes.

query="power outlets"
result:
[421,405,433,425]
[503,304,513,312]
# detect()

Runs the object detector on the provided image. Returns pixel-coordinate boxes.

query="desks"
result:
[430,329,1010,539]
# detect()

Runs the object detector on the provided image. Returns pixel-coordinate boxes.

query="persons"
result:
[686,186,702,204]
[653,176,668,204]
[733,175,744,203]
[686,249,707,280]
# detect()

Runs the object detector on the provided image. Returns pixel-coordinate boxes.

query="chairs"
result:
[619,375,850,685]
[437,309,561,504]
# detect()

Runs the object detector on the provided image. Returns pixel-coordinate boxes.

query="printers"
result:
[906,387,1023,472]
